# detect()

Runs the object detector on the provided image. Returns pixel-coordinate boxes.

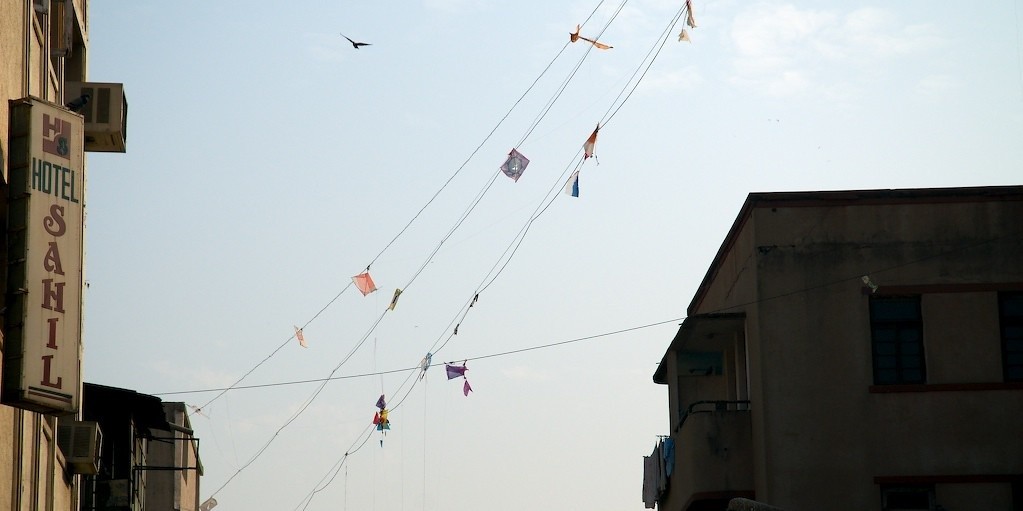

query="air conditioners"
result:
[70,421,103,472]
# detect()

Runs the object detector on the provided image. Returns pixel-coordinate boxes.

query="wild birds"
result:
[340,33,372,49]
